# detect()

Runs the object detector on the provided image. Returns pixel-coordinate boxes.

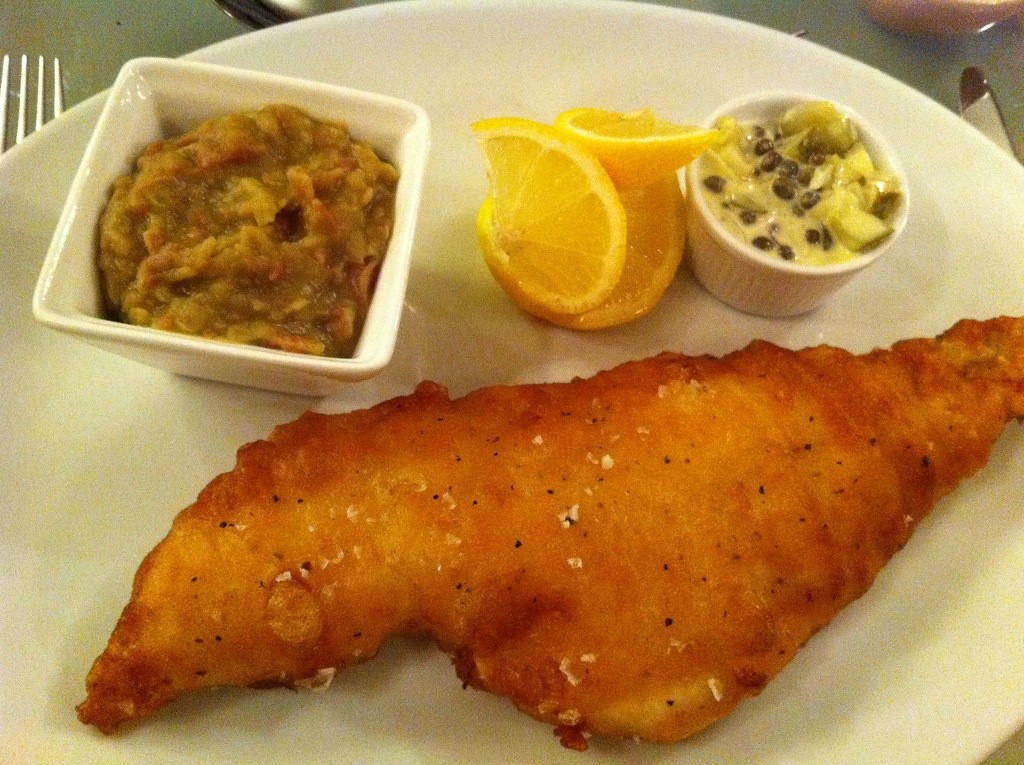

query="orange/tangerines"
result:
[470,106,719,333]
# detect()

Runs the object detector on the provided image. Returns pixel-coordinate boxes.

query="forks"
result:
[0,52,64,153]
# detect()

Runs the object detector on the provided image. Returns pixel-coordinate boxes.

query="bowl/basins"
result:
[32,55,431,398]
[684,88,911,318]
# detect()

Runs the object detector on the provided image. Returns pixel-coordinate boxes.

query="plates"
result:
[0,0,1024,765]
